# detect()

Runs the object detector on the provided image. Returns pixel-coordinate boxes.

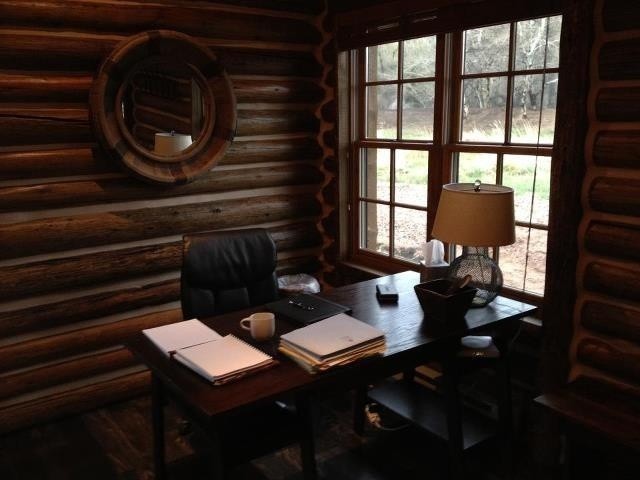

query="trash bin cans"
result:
[360,402,411,476]
[278,273,317,293]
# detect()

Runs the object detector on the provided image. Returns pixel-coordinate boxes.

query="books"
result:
[280,313,385,375]
[170,328,275,386]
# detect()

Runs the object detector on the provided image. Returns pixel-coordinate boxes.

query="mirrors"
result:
[93,32,239,185]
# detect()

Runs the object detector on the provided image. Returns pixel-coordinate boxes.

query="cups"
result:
[240,312,275,341]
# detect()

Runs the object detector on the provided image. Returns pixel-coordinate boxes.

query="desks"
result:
[120,268,542,480]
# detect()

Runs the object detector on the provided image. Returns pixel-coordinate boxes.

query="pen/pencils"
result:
[443,274,472,295]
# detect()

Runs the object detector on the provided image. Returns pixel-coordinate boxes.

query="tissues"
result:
[419,237,453,282]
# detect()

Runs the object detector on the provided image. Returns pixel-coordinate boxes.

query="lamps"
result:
[431,179,517,308]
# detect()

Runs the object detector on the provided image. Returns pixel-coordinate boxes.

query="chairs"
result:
[176,228,281,438]
[366,320,525,480]
